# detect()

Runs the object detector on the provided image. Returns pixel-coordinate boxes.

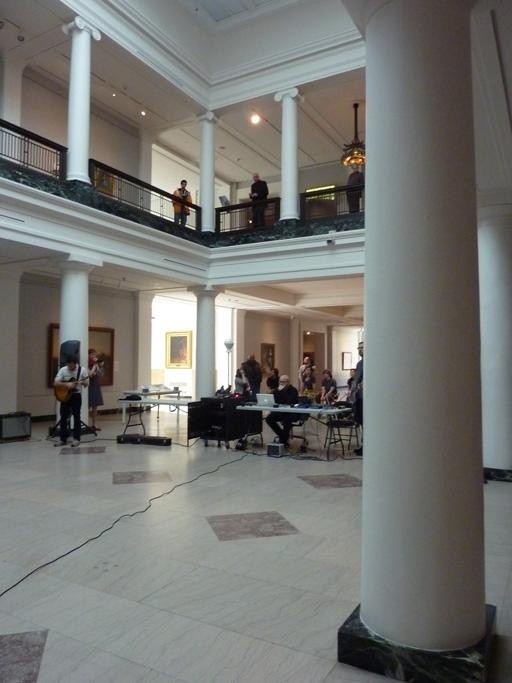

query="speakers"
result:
[267,444,284,457]
[60,340,80,368]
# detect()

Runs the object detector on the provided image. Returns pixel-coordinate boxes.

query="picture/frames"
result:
[260,342,275,372]
[47,323,114,388]
[343,352,352,370]
[166,332,192,369]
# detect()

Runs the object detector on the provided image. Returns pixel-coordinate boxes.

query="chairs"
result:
[324,401,360,450]
[273,396,310,447]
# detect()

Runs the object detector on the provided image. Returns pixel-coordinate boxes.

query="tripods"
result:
[47,414,97,438]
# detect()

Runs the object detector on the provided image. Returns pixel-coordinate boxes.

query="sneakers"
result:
[54,439,81,447]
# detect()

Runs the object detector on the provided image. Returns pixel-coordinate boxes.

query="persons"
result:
[237,342,363,455]
[248,173,269,226]
[88,348,104,431]
[172,180,192,227]
[52,355,88,447]
[345,163,364,214]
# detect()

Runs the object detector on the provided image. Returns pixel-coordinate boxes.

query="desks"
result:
[236,399,351,460]
[122,387,180,424]
[119,398,188,446]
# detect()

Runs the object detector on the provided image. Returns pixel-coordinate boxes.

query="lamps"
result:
[342,104,365,167]
[224,340,234,386]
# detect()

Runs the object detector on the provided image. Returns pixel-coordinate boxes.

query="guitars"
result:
[54,372,96,402]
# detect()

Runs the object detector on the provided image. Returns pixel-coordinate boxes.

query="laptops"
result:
[256,393,276,407]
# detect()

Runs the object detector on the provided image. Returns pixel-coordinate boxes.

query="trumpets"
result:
[93,359,104,366]
